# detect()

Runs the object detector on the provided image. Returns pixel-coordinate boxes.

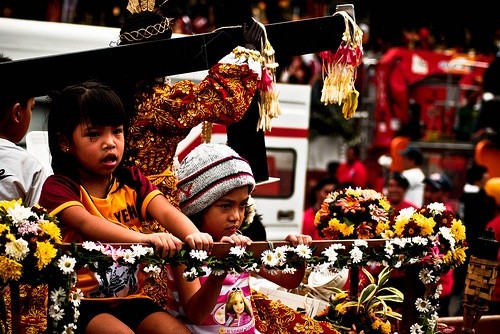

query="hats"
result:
[398,146,424,165]
[176,143,256,215]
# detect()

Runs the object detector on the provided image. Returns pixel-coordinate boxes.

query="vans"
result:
[0,18,311,247]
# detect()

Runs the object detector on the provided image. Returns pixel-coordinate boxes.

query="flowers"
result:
[0,184,469,334]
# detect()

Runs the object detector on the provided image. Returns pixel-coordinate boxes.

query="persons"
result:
[0,53,47,210]
[303,144,500,318]
[166,142,312,334]
[39,81,214,333]
